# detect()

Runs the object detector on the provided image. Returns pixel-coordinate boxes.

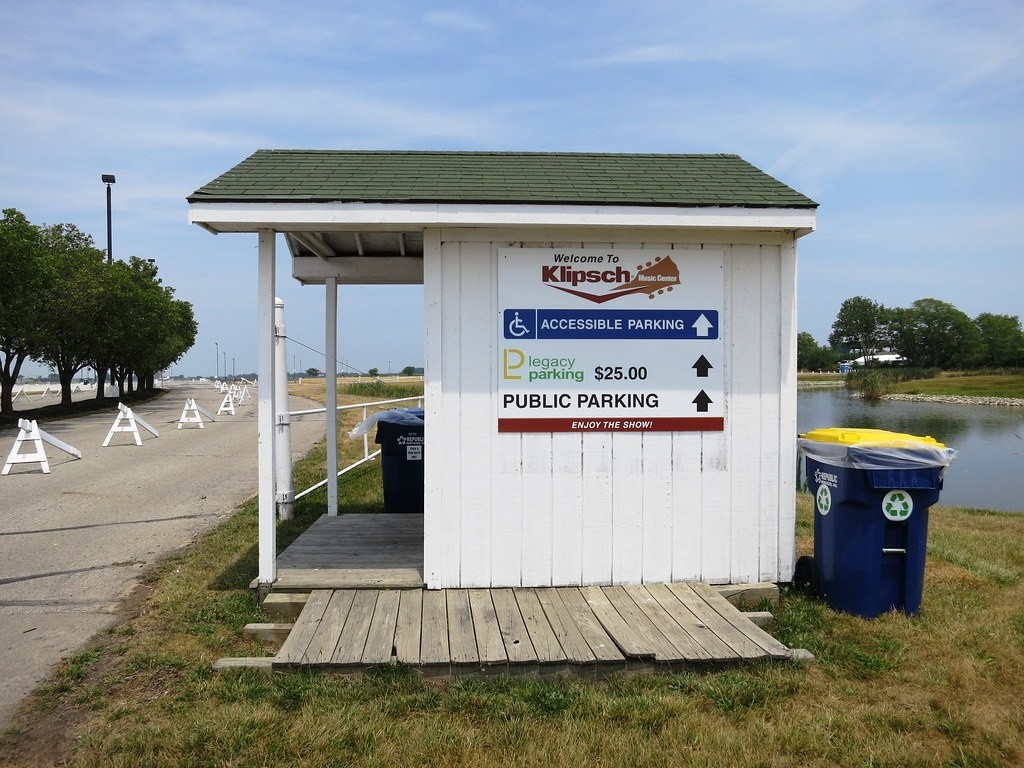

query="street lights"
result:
[100,173,117,387]
[215,342,219,379]
[232,357,234,375]
[224,352,226,378]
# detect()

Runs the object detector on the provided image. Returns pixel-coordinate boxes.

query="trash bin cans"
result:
[375,408,424,514]
[798,427,957,618]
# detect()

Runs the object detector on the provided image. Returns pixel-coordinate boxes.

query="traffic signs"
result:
[498,306,725,432]
